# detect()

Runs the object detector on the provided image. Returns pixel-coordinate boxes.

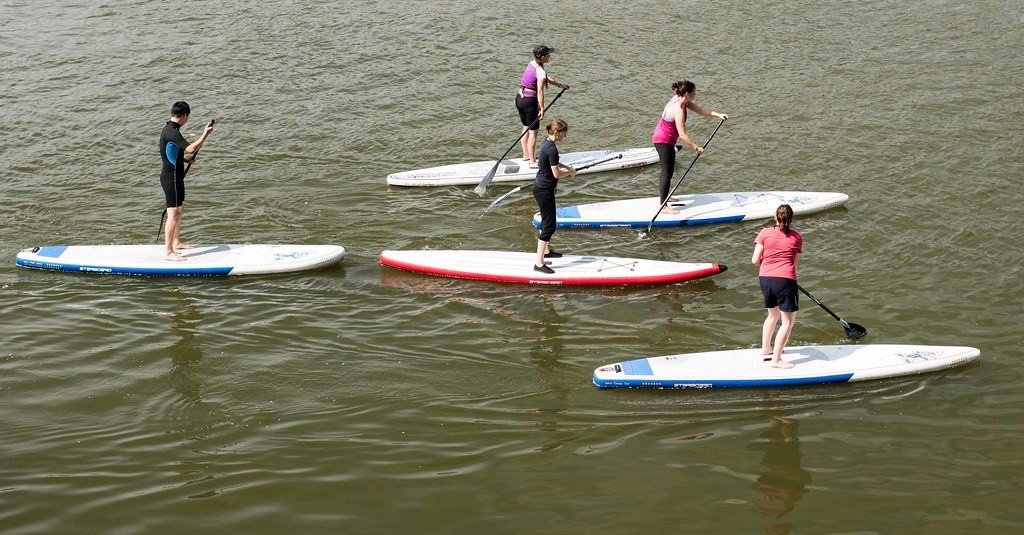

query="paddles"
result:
[155,118,216,241]
[490,153,624,208]
[798,285,868,340]
[477,85,569,189]
[646,115,727,234]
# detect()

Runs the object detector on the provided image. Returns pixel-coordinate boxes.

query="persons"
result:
[652,80,728,215]
[515,45,569,168]
[159,100,213,260]
[532,119,576,273]
[751,205,803,370]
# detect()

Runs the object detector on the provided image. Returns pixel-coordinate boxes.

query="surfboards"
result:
[385,143,683,187]
[14,243,348,279]
[531,190,850,231]
[377,248,730,287]
[591,343,983,391]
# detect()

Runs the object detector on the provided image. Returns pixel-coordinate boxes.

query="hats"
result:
[533,45,555,58]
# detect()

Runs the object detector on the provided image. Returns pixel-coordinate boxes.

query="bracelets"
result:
[558,84,562,87]
[695,146,698,150]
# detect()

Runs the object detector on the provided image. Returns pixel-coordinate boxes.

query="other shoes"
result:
[544,250,562,258]
[534,264,555,274]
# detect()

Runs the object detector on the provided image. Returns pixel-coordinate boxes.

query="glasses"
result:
[185,114,190,120]
